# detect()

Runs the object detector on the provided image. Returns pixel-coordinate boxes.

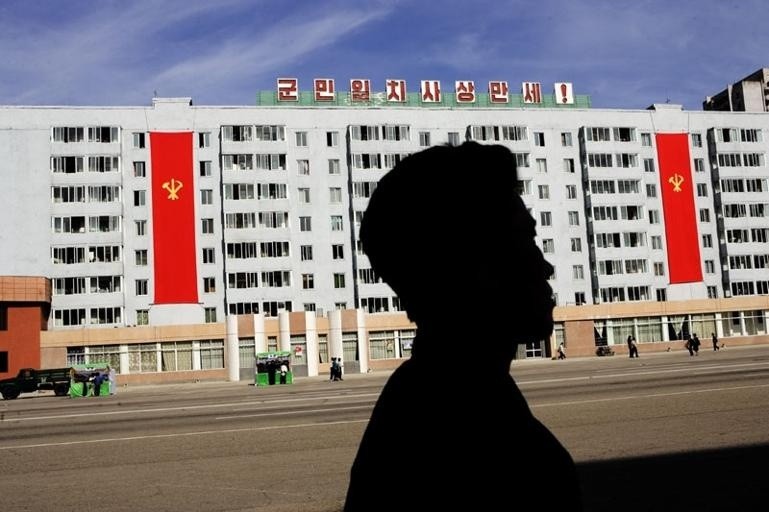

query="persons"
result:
[630,337,639,357]
[75,370,107,397]
[341,139,589,511]
[329,357,344,382]
[711,332,719,352]
[557,342,566,360]
[258,358,290,384]
[692,333,701,356]
[627,335,632,350]
[686,334,695,356]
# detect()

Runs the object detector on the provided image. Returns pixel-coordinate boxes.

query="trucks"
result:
[0,366,77,400]
